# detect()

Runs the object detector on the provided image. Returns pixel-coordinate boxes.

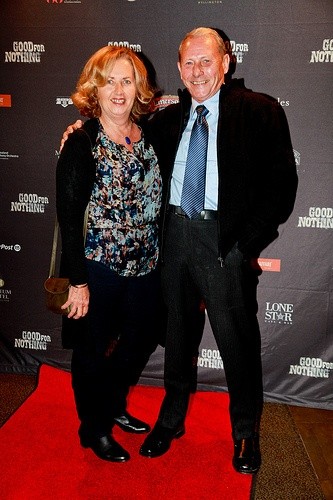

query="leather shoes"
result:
[138,423,185,457]
[232,432,261,473]
[76,429,130,462]
[113,413,150,433]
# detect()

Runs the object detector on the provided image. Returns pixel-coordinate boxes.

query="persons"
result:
[55,46,167,461]
[58,27,301,474]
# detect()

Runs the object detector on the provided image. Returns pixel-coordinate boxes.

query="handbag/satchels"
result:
[43,276,73,316]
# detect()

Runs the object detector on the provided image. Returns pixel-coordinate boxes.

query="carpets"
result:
[0,365,258,500]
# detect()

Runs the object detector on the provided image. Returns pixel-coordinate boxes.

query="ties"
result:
[179,105,209,220]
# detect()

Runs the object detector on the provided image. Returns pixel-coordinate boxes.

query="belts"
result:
[166,204,219,219]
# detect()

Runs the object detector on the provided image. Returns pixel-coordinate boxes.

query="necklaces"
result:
[104,116,136,145]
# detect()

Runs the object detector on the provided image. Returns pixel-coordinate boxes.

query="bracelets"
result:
[70,283,88,290]
